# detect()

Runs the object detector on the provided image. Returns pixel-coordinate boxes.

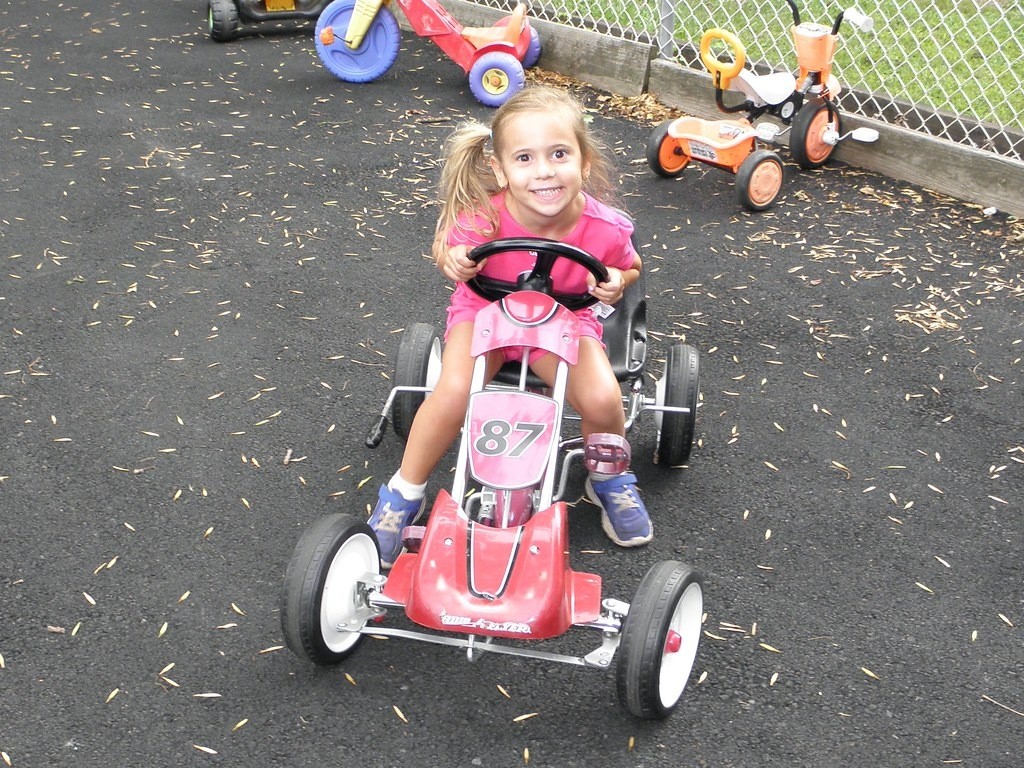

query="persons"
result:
[365,86,656,568]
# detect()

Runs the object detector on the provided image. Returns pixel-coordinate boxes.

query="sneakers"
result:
[585,466,654,547]
[367,482,426,568]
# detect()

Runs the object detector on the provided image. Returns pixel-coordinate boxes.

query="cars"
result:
[281,231,709,723]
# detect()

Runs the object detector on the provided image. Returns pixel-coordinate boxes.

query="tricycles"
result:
[314,0,543,108]
[647,1,875,214]
